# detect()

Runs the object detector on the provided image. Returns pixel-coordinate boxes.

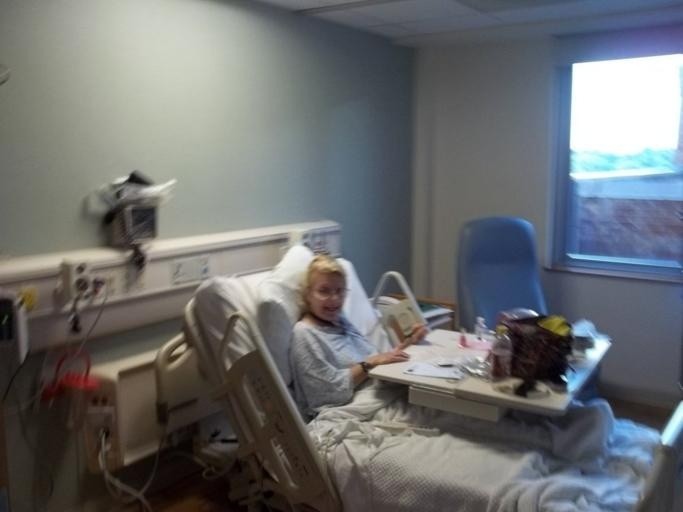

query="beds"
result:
[184,247,682,511]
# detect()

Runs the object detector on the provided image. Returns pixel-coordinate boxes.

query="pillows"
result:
[257,245,319,386]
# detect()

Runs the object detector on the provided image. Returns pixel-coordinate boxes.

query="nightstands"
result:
[387,293,459,330]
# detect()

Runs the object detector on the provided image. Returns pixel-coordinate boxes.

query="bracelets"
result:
[358,359,370,376]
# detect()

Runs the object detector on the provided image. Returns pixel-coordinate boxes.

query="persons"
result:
[288,254,430,420]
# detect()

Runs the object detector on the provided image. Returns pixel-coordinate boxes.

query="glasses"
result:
[314,287,351,297]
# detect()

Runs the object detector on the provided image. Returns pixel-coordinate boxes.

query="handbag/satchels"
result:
[495,309,571,376]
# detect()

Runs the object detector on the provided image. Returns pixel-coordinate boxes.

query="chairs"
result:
[456,214,545,329]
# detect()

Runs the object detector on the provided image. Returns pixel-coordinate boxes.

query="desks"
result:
[368,328,611,423]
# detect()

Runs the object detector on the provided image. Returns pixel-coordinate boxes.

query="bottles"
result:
[489,325,513,382]
